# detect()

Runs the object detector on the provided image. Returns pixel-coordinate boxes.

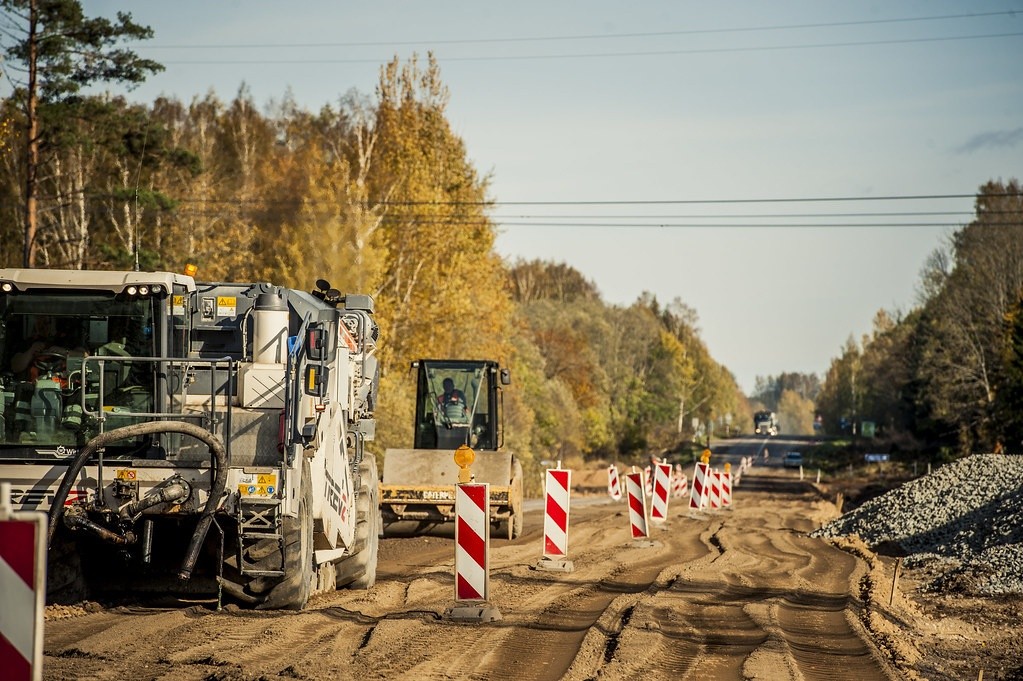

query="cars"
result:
[783,453,804,469]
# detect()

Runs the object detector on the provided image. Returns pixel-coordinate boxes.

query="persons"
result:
[436,378,472,424]
[13,314,91,438]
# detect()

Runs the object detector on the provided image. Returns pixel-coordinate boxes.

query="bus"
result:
[753,409,777,437]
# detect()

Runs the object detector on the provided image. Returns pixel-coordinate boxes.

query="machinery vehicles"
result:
[377,358,524,542]
[0,261,384,616]
